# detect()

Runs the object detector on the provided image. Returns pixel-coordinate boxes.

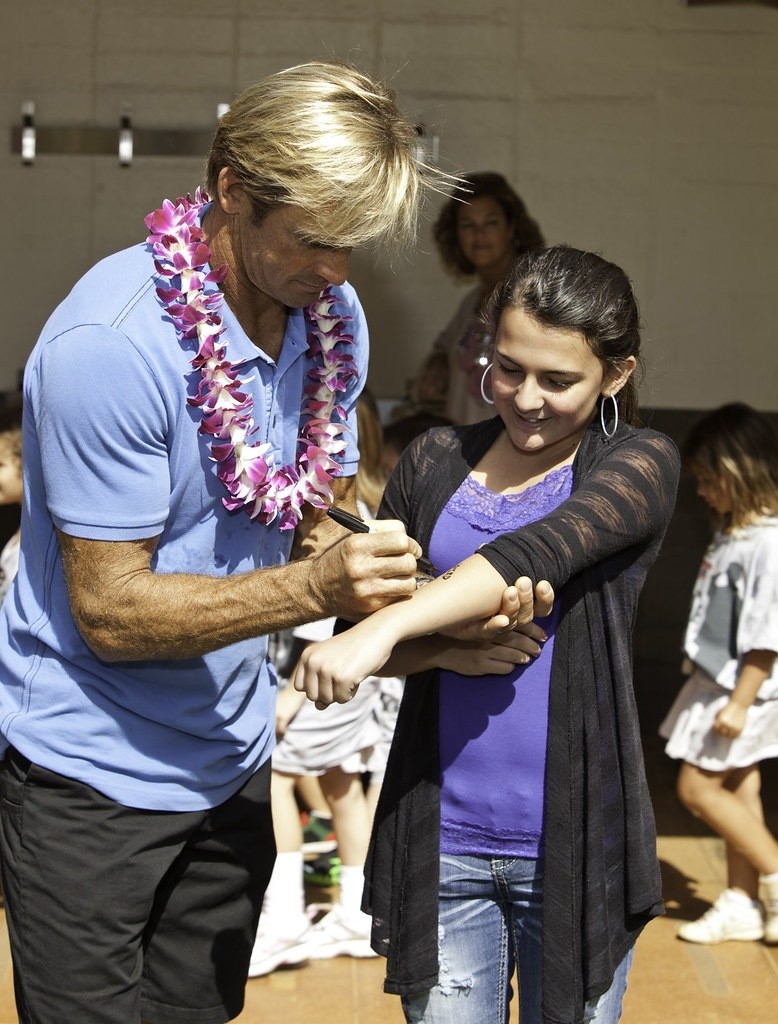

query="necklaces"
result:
[143,184,354,524]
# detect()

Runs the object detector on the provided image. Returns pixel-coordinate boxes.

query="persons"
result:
[355,386,399,523]
[291,246,681,1024]
[0,431,26,599]
[249,506,401,978]
[660,400,778,945]
[400,171,546,424]
[0,61,562,1024]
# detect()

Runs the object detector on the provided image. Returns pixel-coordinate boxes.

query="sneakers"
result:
[760,874,778,943]
[678,890,765,945]
[310,900,380,957]
[248,912,315,977]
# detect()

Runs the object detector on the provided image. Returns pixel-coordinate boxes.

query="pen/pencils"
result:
[328,502,442,583]
